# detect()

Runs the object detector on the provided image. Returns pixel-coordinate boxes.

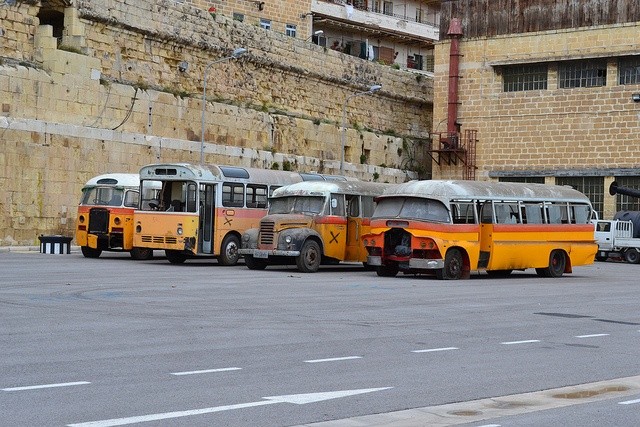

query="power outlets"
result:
[590,218,640,262]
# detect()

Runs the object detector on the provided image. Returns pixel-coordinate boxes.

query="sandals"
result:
[133,164,358,267]
[238,180,395,272]
[361,180,599,279]
[76,174,140,257]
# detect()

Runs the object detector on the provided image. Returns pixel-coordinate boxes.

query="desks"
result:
[339,84,381,175]
[200,48,245,162]
[306,30,324,43]
